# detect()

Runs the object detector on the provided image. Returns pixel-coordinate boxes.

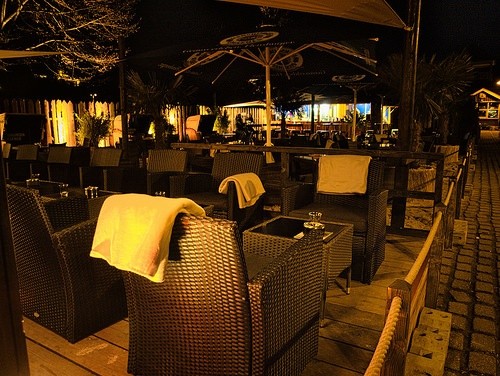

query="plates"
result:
[304,222,325,229]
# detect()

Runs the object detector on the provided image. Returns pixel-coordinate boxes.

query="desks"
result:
[242,215,353,329]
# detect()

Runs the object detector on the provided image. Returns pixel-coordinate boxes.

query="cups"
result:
[309,212,322,226]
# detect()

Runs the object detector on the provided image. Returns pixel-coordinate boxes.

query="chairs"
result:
[6,122,398,376]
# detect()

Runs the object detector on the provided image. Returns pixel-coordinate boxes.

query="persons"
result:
[228,103,361,135]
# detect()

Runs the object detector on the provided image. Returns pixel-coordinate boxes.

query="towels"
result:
[316,154,372,195]
[219,172,266,209]
[89,193,206,283]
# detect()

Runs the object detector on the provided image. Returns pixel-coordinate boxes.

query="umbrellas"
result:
[308,73,381,141]
[174,24,380,164]
[0,0,413,166]
